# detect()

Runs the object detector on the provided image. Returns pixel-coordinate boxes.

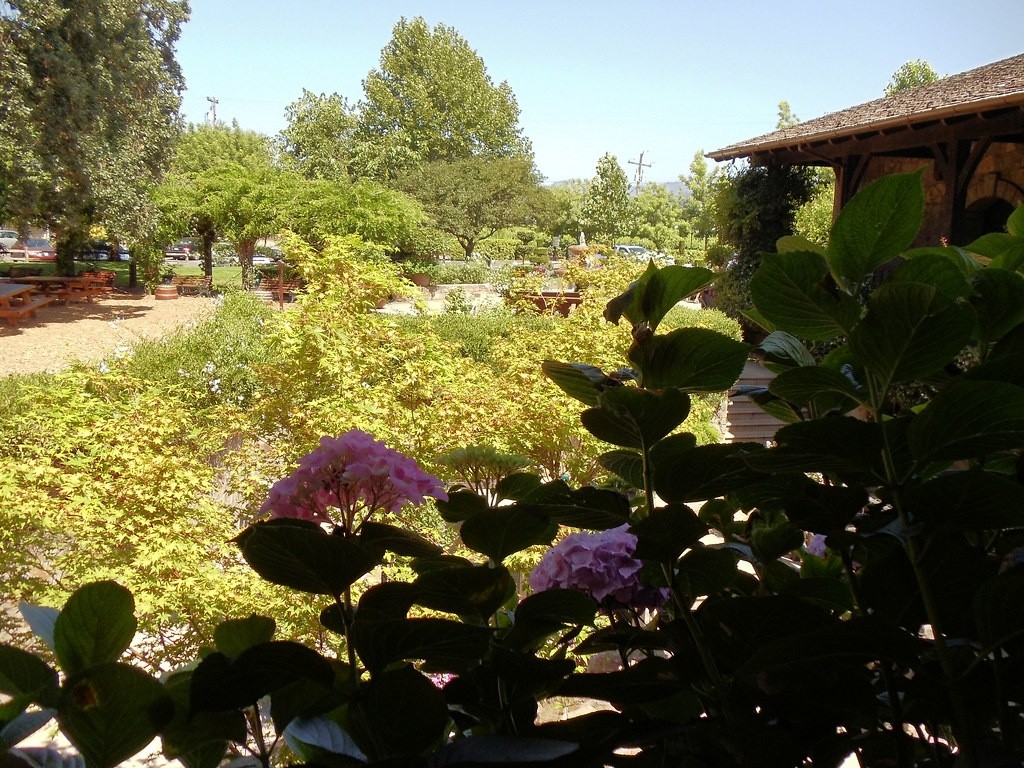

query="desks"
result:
[11,275,101,308]
[0,283,36,328]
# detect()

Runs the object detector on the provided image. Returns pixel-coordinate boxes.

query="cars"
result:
[9,238,57,262]
[80,240,133,262]
[165,236,205,260]
[614,246,648,257]
[0,229,23,249]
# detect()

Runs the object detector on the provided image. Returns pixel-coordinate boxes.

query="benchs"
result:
[249,277,302,303]
[80,269,116,297]
[169,275,213,298]
[61,289,100,307]
[13,296,54,322]
[515,289,584,318]
[247,291,274,306]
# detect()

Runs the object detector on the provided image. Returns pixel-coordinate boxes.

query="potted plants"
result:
[154,264,179,300]
[404,261,438,287]
